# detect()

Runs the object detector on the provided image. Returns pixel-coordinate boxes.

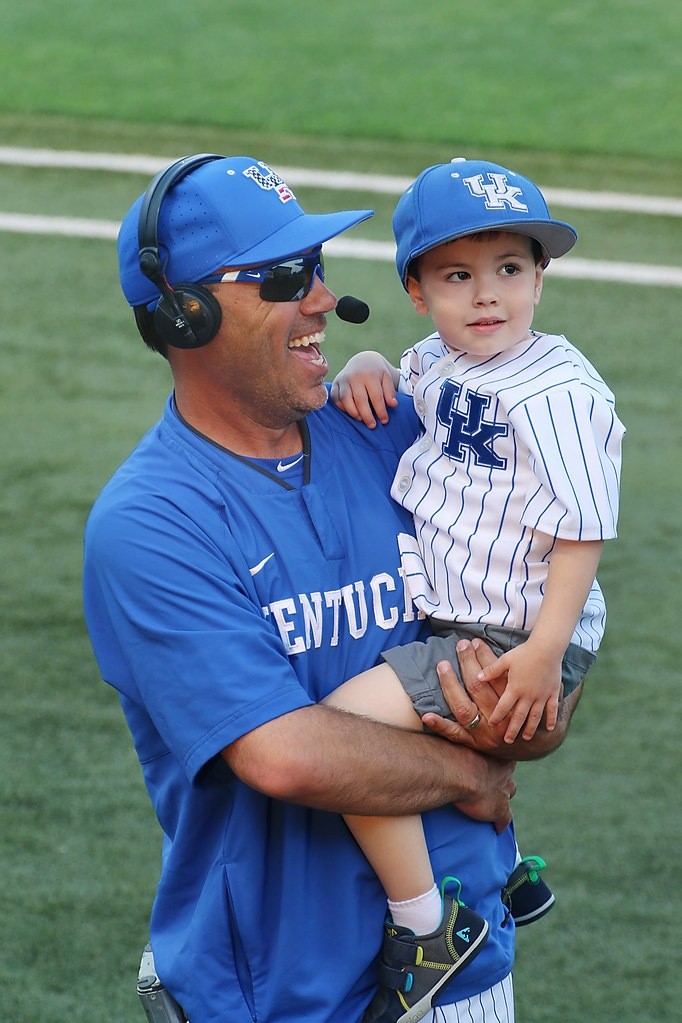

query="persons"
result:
[79,153,586,1023]
[318,152,627,1023]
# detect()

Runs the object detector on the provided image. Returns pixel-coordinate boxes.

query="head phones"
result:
[137,154,225,350]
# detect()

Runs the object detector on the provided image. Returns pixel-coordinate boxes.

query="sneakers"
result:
[501,856,555,928]
[363,876,490,1023]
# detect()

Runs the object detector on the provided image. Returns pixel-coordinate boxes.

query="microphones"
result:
[336,296,370,324]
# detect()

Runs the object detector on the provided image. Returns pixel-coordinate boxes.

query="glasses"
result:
[192,245,325,302]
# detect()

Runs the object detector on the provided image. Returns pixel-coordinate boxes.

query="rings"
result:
[465,712,481,730]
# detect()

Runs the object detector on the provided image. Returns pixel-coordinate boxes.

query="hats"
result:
[393,157,577,293]
[117,156,375,308]
